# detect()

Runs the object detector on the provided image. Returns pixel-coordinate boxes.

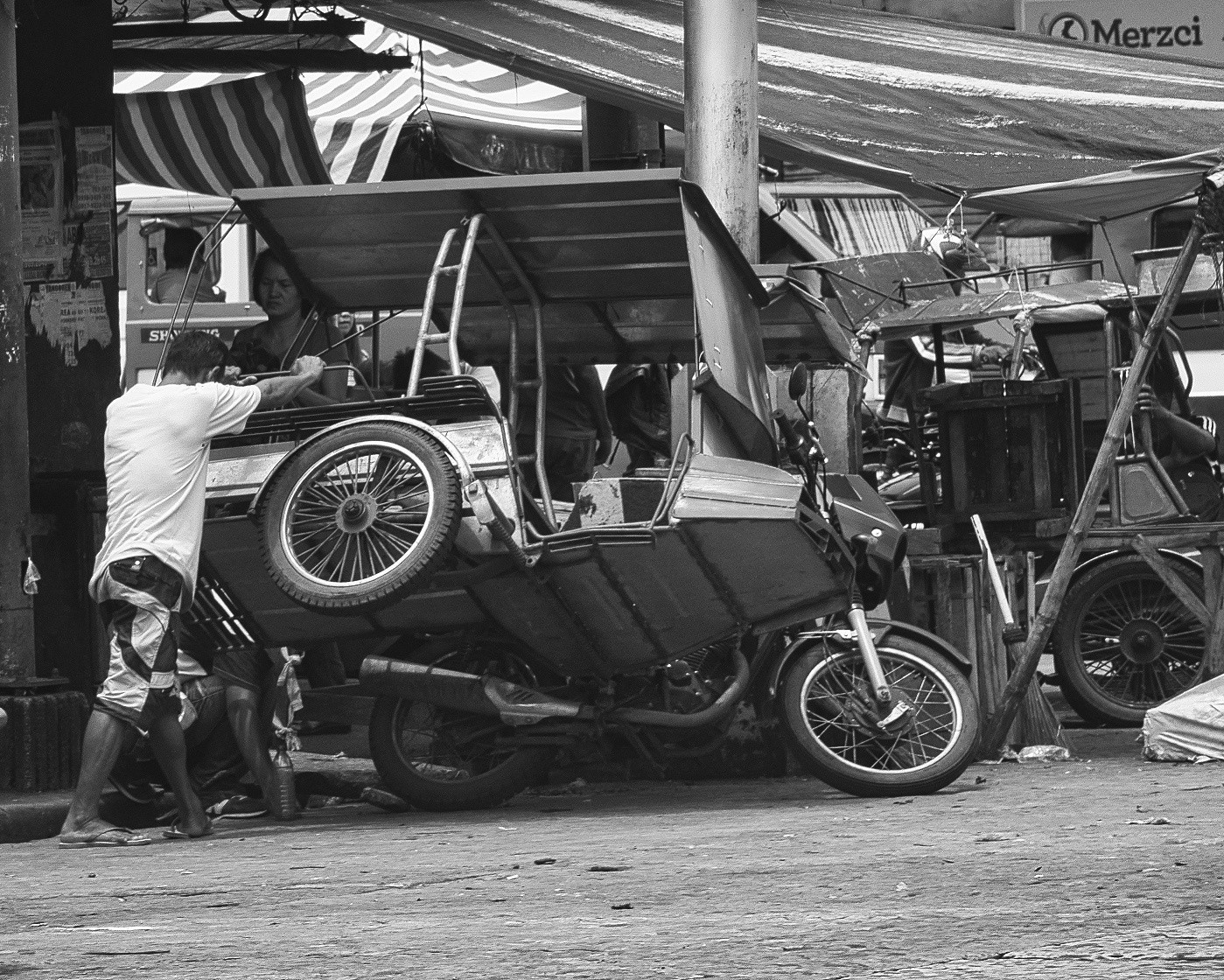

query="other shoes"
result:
[289,718,351,735]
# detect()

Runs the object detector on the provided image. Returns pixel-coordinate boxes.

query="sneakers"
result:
[202,789,271,823]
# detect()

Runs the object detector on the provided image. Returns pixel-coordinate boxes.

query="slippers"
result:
[58,826,152,849]
[162,814,214,838]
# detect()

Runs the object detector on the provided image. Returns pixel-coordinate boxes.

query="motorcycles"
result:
[114,141,1223,800]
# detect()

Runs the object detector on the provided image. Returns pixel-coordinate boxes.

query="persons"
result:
[320,311,362,384]
[1120,377,1223,486]
[493,364,611,500]
[390,348,501,410]
[60,331,326,849]
[151,229,226,302]
[876,226,1040,424]
[210,642,343,809]
[603,362,680,474]
[229,248,348,406]
